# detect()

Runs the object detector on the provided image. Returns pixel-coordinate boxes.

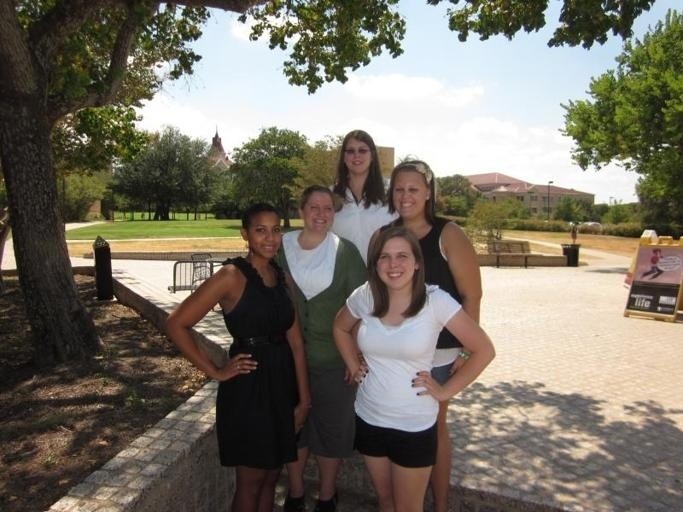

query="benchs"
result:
[487,240,537,269]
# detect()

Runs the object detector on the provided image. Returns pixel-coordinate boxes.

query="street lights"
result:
[547,181,554,220]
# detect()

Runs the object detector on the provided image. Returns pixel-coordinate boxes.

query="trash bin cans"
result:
[561,244,580,266]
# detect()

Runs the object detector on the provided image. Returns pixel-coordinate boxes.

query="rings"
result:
[236,364,240,369]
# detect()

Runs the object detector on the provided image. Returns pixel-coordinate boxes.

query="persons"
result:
[161,203,312,511]
[272,188,368,511]
[333,226,495,511]
[328,129,400,268]
[384,155,483,512]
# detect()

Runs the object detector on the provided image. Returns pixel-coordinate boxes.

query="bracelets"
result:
[456,350,471,360]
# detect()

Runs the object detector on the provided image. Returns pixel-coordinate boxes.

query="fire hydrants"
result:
[91,236,117,304]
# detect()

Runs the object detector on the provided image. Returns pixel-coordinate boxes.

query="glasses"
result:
[342,148,371,156]
